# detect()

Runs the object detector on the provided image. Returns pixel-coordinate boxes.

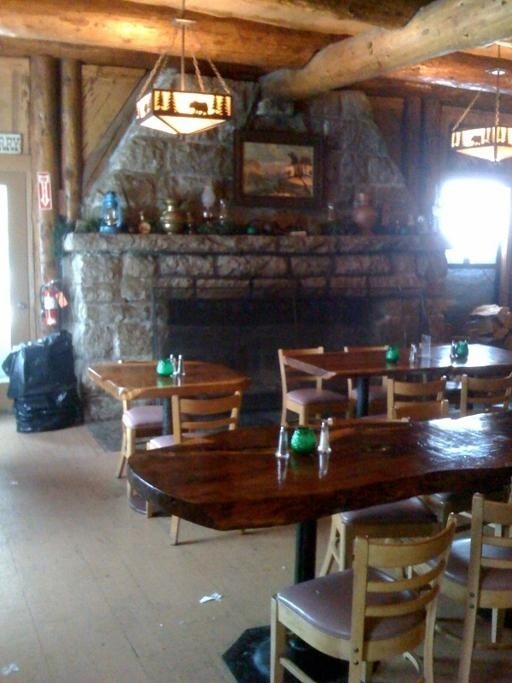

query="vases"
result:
[158,197,186,235]
[350,190,380,237]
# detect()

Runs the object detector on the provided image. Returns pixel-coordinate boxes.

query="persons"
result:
[280,151,312,188]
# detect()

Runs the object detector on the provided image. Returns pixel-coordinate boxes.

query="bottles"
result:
[275,425,291,459]
[176,354,185,375]
[168,354,176,375]
[277,459,288,485]
[317,419,332,453]
[171,376,184,386]
[457,339,467,357]
[450,340,457,357]
[386,344,400,362]
[409,342,422,361]
[318,453,330,479]
[292,423,317,455]
[156,355,173,377]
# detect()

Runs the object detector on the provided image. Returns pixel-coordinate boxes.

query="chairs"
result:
[387,377,449,420]
[408,490,511,681]
[344,345,393,418]
[146,392,246,547]
[267,511,456,683]
[319,414,435,577]
[278,345,350,426]
[460,371,511,413]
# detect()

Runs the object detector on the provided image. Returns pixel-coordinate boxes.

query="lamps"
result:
[95,189,124,237]
[199,181,215,225]
[444,39,511,163]
[130,0,242,140]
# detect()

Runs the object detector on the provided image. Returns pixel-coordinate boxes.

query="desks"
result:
[86,359,249,520]
[124,404,511,683]
[285,343,511,417]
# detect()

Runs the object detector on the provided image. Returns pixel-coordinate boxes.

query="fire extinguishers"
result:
[40,280,60,327]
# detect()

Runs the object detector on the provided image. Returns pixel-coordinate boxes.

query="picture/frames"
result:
[227,123,330,212]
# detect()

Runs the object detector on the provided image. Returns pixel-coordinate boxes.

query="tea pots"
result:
[97,188,124,234]
